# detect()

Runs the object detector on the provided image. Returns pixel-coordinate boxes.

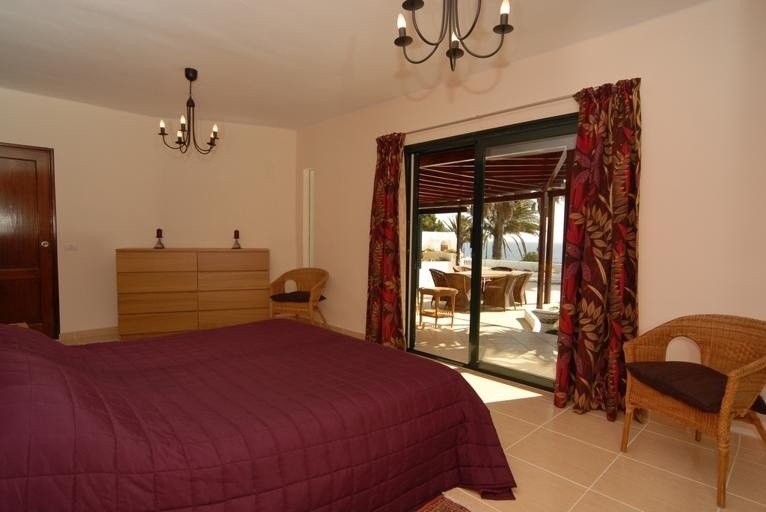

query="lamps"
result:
[394,0,513,71]
[158,68,219,154]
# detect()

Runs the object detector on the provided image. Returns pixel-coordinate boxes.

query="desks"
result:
[419,287,458,329]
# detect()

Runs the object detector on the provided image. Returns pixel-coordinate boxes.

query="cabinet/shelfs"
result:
[116,248,270,340]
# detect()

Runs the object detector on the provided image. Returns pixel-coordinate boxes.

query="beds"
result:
[0,322,517,512]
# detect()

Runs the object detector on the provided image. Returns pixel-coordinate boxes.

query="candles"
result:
[157,229,162,238]
[234,229,239,239]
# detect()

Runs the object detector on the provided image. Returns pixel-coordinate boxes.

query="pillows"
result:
[270,291,326,303]
[625,361,766,413]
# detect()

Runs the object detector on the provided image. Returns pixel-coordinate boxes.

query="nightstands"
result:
[9,323,29,328]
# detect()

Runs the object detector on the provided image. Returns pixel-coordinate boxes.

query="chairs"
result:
[429,266,534,313]
[270,267,327,326]
[620,314,766,509]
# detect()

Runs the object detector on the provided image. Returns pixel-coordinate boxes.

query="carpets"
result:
[416,495,471,512]
